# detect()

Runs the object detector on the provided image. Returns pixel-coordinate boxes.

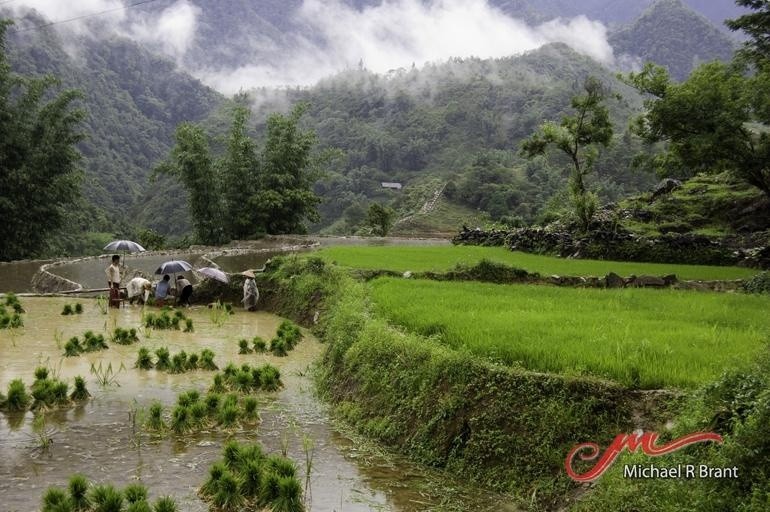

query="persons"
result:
[174,275,193,307]
[108,255,121,310]
[125,277,152,306]
[154,274,177,307]
[239,269,259,310]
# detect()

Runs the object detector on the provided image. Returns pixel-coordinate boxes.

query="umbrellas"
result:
[155,258,193,284]
[197,266,230,293]
[103,240,146,268]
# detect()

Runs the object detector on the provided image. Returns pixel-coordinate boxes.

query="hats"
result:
[144,281,151,289]
[242,270,255,278]
